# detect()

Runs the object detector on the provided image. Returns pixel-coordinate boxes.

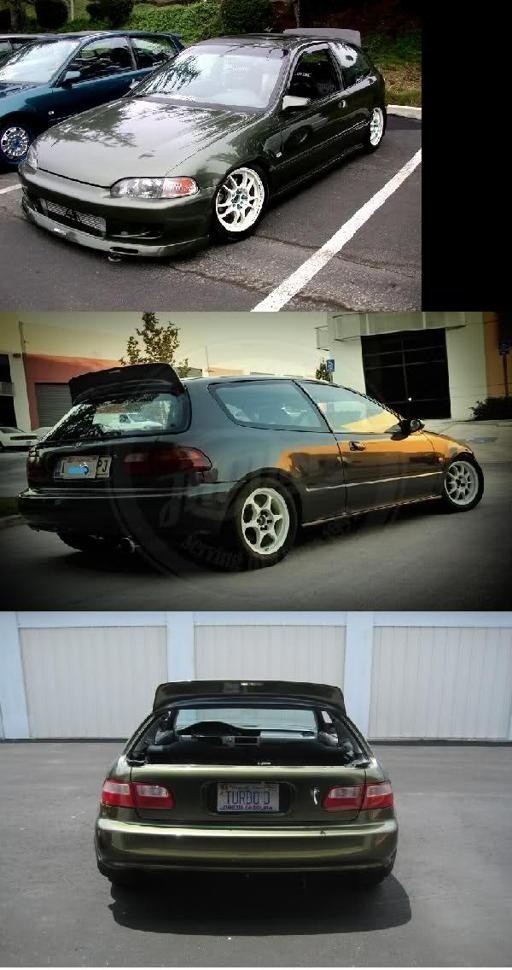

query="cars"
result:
[95,680,398,884]
[0,29,183,171]
[0,427,37,451]
[17,27,387,262]
[17,363,484,567]
